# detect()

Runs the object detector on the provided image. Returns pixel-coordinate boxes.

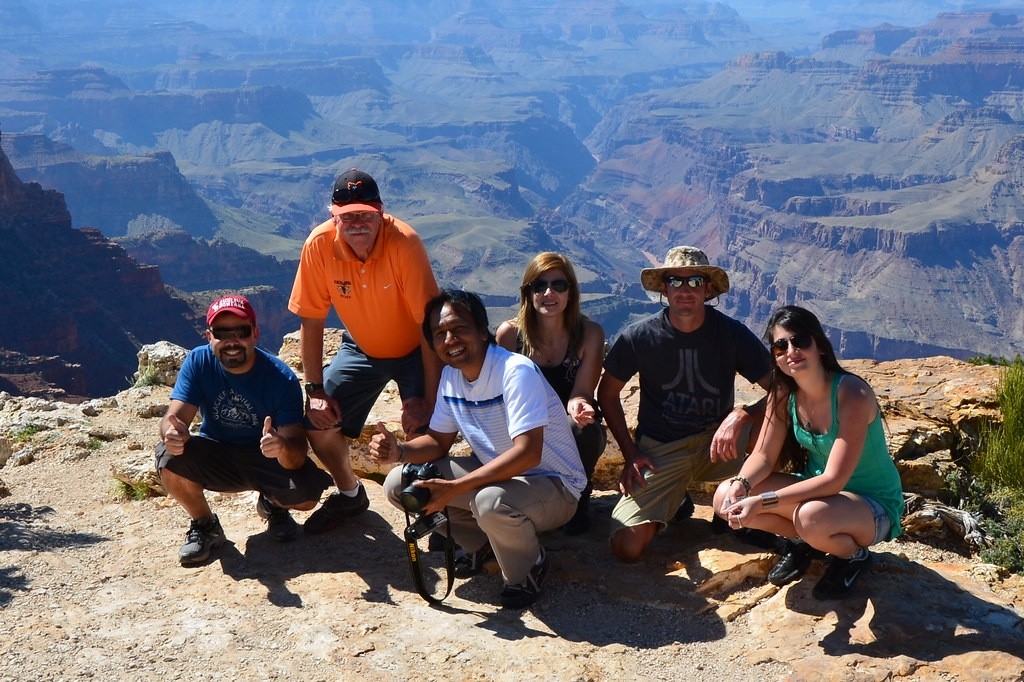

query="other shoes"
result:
[677,489,696,520]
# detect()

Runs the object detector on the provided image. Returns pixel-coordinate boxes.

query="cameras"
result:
[398,462,444,513]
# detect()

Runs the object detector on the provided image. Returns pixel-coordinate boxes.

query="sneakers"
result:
[812,549,867,599]
[502,546,548,609]
[304,480,370,534]
[454,549,490,577]
[256,494,298,542]
[179,513,227,564]
[768,545,826,585]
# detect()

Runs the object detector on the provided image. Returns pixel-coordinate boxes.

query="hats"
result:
[331,167,384,215]
[641,246,729,303]
[206,295,256,325]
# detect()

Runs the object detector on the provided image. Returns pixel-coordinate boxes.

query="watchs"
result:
[305,382,323,393]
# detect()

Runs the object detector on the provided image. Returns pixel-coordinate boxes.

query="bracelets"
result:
[397,443,406,462]
[730,476,752,497]
[762,491,779,509]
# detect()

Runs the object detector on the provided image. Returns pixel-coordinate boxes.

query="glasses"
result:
[663,275,707,287]
[772,334,812,355]
[208,325,252,339]
[531,280,572,294]
[339,211,379,222]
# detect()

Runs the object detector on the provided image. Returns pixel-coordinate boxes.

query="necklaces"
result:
[536,336,563,363]
[805,393,820,430]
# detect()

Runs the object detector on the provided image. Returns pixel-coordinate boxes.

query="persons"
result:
[496,251,607,537]
[288,167,447,535]
[369,288,588,611]
[597,245,775,561]
[155,294,324,564]
[713,305,904,601]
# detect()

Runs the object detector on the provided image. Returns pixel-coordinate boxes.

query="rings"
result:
[736,515,740,522]
[739,522,743,528]
